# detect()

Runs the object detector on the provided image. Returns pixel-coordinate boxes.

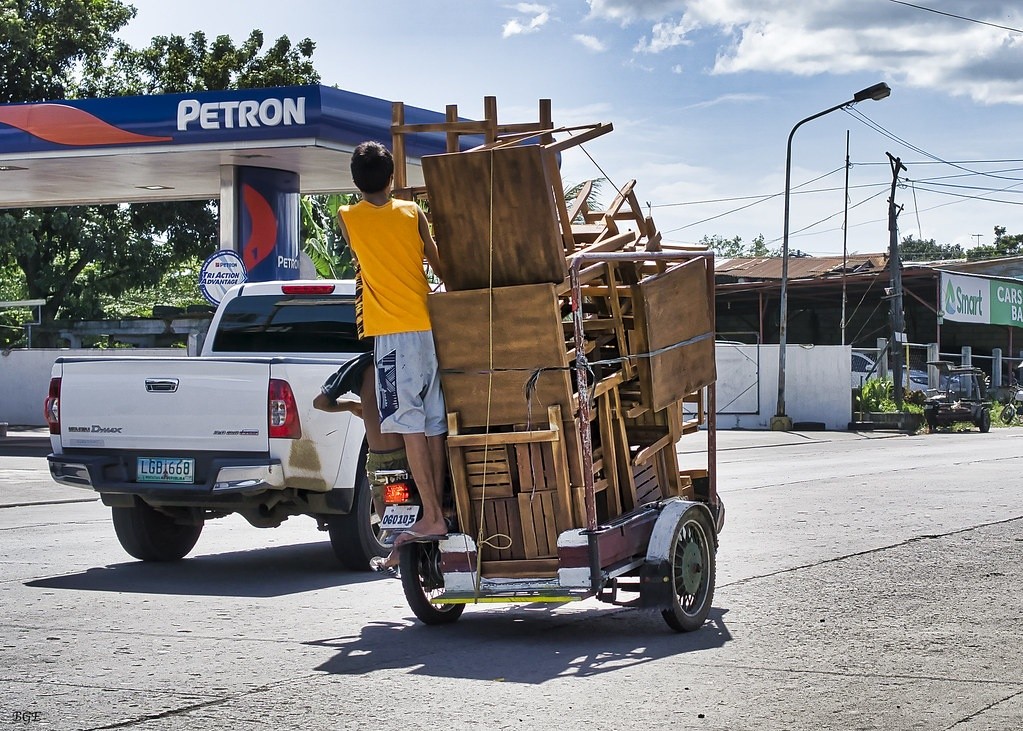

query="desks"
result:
[390,97,716,574]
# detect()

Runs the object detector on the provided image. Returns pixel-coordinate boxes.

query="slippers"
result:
[393,530,449,547]
[370,556,402,579]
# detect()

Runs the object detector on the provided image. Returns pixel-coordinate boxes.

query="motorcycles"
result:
[1000,378,1023,424]
[365,254,724,633]
[922,361,993,433]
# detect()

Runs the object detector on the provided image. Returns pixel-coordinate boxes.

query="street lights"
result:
[774,81,892,418]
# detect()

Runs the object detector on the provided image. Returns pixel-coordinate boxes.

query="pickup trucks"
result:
[42,280,394,575]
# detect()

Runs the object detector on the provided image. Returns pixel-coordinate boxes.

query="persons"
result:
[312,141,448,578]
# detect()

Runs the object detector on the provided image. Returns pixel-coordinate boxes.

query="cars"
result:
[851,351,961,406]
